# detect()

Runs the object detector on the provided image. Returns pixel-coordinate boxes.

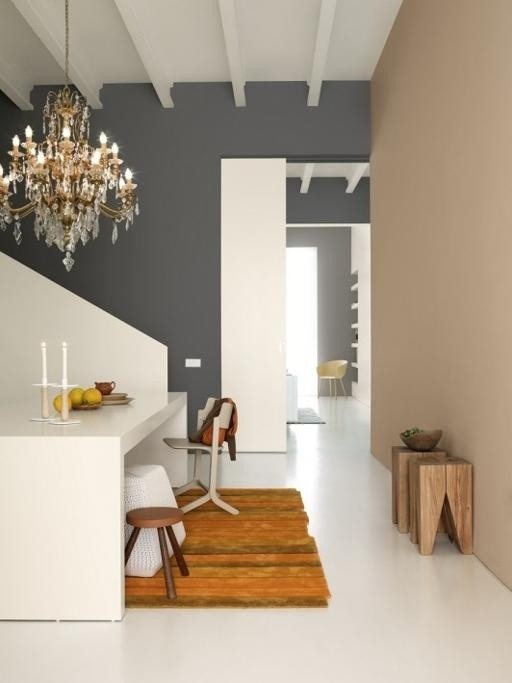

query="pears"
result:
[53,395,72,413]
[82,388,102,406]
[68,388,85,409]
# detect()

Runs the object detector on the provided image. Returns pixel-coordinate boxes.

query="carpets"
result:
[287,406,326,424]
[124,487,332,608]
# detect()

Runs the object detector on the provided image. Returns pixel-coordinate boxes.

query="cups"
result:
[94,381,116,395]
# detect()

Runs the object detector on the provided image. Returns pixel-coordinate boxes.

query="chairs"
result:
[316,359,348,400]
[163,396,241,516]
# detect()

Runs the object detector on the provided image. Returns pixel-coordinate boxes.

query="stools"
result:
[124,506,190,594]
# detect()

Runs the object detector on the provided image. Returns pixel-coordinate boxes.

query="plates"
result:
[103,398,133,405]
[101,393,128,401]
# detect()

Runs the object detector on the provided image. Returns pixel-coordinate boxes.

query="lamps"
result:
[2,0,143,271]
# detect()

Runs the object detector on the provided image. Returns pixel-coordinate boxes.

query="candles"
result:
[39,341,49,383]
[61,341,70,383]
